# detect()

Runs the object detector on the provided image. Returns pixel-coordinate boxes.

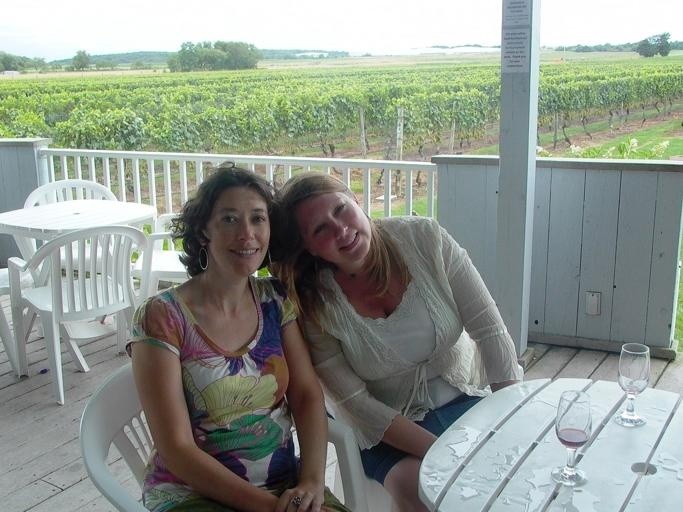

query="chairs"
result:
[0,278,19,375]
[80,360,369,511]
[6,220,151,407]
[20,177,124,286]
[131,212,259,302]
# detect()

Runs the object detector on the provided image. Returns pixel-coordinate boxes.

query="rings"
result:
[290,496,302,504]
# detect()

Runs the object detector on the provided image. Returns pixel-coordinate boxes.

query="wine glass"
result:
[550,390,594,487]
[612,343,651,427]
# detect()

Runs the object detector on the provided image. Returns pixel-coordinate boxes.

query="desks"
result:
[416,378,681,512]
[1,199,159,373]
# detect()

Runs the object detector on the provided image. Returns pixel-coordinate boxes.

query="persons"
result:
[266,170,523,512]
[124,161,353,512]
